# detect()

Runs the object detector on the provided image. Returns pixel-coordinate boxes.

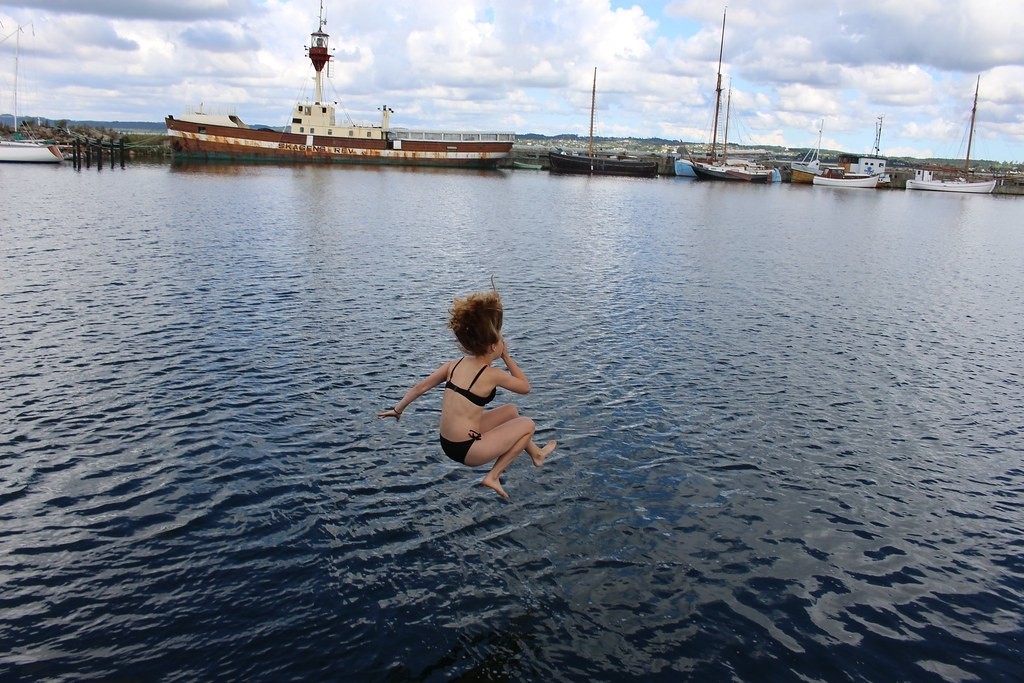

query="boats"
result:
[513,162,542,170]
[164,0,514,170]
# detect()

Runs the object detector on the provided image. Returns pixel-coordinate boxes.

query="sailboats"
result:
[675,5,775,183]
[548,67,659,179]
[791,116,892,191]
[905,74,998,194]
[0,19,87,164]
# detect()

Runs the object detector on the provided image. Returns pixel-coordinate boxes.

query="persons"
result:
[376,289,558,499]
[840,170,845,179]
[826,169,832,177]
